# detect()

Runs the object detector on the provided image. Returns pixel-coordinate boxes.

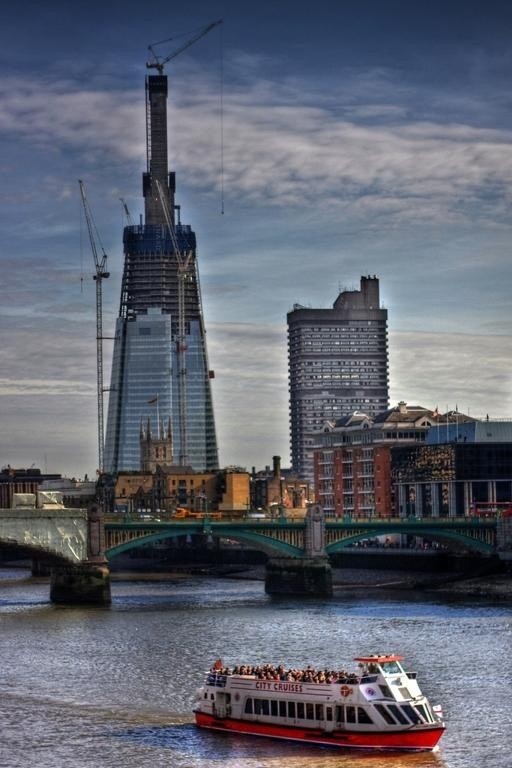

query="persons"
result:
[210,658,369,684]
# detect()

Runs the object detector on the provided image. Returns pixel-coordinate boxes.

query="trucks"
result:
[172,507,223,520]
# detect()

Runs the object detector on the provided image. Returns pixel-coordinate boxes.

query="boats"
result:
[191,653,448,751]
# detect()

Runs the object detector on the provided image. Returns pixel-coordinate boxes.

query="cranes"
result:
[141,15,227,80]
[71,173,115,505]
[154,179,198,465]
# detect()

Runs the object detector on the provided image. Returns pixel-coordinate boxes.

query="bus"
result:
[469,500,512,519]
